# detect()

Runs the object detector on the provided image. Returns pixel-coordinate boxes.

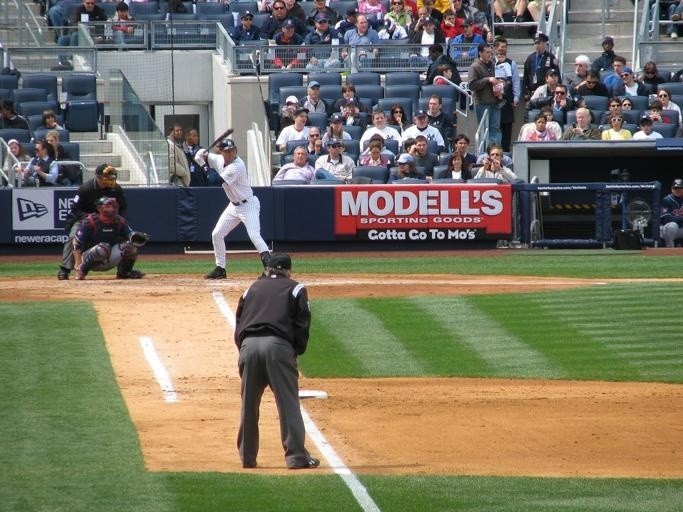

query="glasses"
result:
[543,57,666,122]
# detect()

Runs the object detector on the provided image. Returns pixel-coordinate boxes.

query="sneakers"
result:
[117,270,146,279]
[260,251,270,268]
[671,12,680,20]
[57,270,70,280]
[206,266,225,279]
[304,458,320,467]
[670,32,678,39]
[75,271,85,280]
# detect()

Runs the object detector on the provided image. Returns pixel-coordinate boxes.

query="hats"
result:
[116,2,128,10]
[268,251,291,269]
[285,81,431,166]
[238,4,488,29]
[671,180,683,189]
[531,32,548,43]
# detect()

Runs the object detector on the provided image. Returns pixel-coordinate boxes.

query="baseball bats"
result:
[203,128,232,155]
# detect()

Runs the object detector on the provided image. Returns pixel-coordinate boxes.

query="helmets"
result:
[95,164,117,190]
[219,139,236,151]
[93,196,119,217]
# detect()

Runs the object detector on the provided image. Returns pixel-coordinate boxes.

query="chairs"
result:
[266,70,681,182]
[0,70,103,189]
[44,0,429,69]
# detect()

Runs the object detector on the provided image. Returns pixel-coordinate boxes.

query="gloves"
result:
[194,154,205,167]
[196,148,208,157]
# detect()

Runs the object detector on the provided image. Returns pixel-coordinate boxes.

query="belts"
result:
[231,193,254,206]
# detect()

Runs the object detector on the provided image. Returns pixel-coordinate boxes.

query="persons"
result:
[234,251,319,468]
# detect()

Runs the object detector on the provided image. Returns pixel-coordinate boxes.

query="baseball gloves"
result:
[129,232,150,248]
